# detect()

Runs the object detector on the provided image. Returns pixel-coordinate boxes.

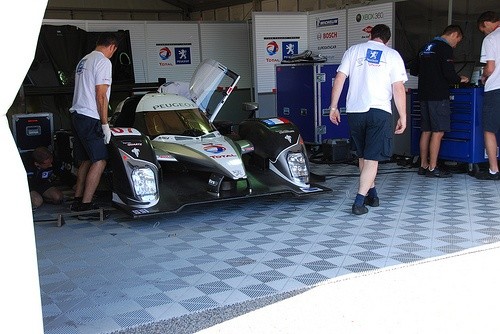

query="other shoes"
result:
[352,205,368,215]
[475,169,500,180]
[419,164,429,174]
[366,196,379,207]
[70,202,111,220]
[427,167,452,178]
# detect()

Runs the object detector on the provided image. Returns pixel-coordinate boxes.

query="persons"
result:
[328,24,407,214]
[410,24,470,178]
[28,146,64,207]
[70,33,119,221]
[479,11,500,180]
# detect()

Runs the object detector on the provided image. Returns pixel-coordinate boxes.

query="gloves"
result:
[102,124,112,145]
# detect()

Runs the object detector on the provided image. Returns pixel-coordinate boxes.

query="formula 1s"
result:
[50,58,334,219]
